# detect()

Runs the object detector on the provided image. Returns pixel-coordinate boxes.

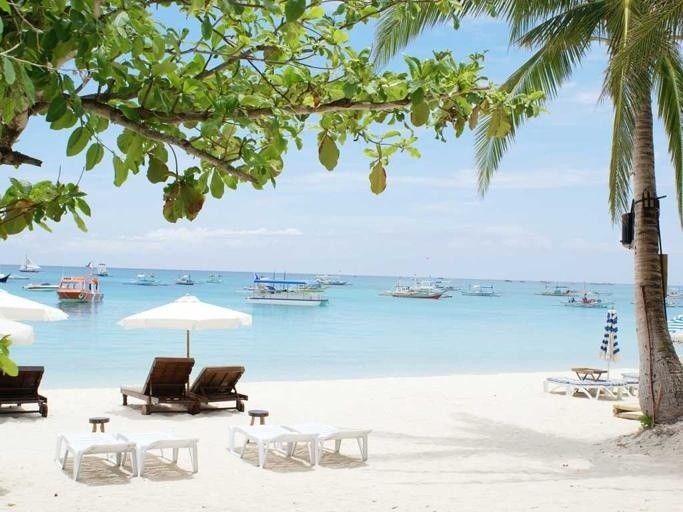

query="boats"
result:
[244,270,329,307]
[7,274,29,280]
[0,272,10,283]
[233,269,351,292]
[86,258,224,286]
[55,273,104,304]
[22,281,58,292]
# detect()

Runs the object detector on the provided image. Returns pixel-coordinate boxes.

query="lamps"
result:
[618,192,665,250]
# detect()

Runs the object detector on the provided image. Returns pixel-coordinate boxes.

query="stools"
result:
[246,409,268,425]
[87,417,109,432]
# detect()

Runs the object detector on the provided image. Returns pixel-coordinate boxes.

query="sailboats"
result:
[18,253,41,273]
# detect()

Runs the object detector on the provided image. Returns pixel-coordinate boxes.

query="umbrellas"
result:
[0,289,70,323]
[0,320,34,346]
[599,305,621,378]
[114,294,253,395]
[666,314,683,345]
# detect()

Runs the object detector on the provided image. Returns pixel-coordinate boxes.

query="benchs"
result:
[544,368,639,401]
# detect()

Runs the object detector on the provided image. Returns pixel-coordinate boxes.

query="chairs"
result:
[53,432,137,482]
[289,422,371,462]
[187,366,247,415]
[119,357,198,416]
[229,426,314,470]
[133,434,199,476]
[0,365,47,417]
